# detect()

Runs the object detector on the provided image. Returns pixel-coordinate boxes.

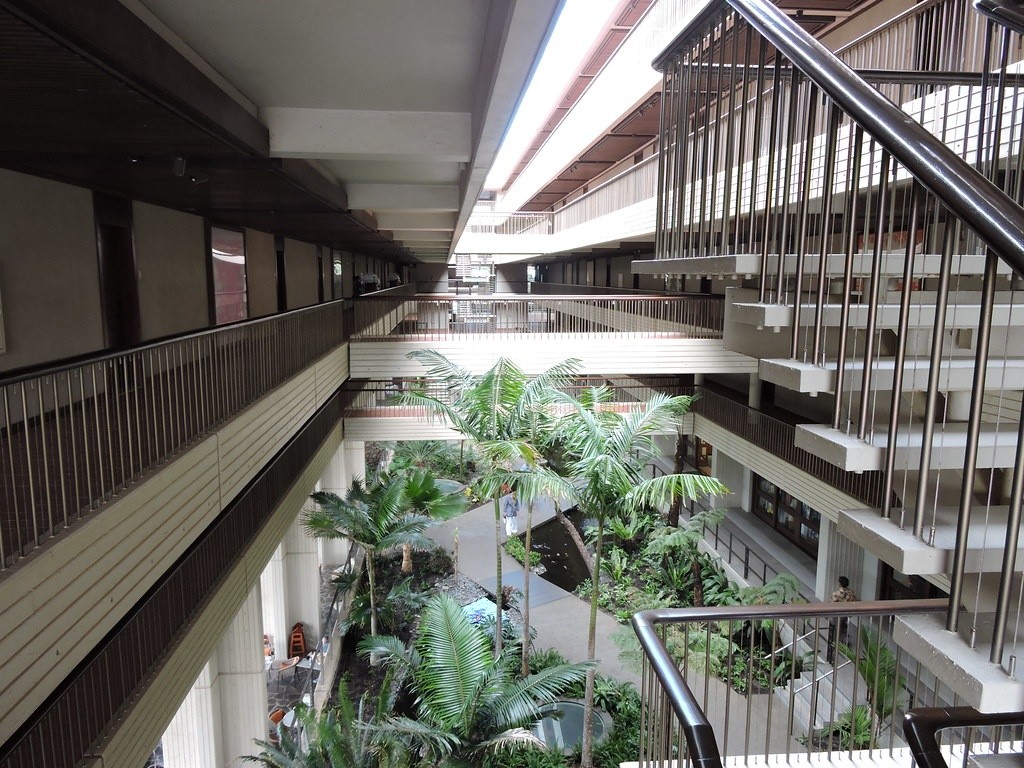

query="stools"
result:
[290,631,305,658]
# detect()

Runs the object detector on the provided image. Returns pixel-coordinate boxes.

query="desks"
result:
[265,655,276,685]
[296,652,322,683]
[283,708,302,728]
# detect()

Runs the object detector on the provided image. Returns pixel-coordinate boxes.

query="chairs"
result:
[268,708,286,724]
[302,693,310,707]
[277,656,300,683]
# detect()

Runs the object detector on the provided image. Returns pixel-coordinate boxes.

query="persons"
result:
[502,490,520,538]
[827,576,858,666]
[316,636,332,657]
